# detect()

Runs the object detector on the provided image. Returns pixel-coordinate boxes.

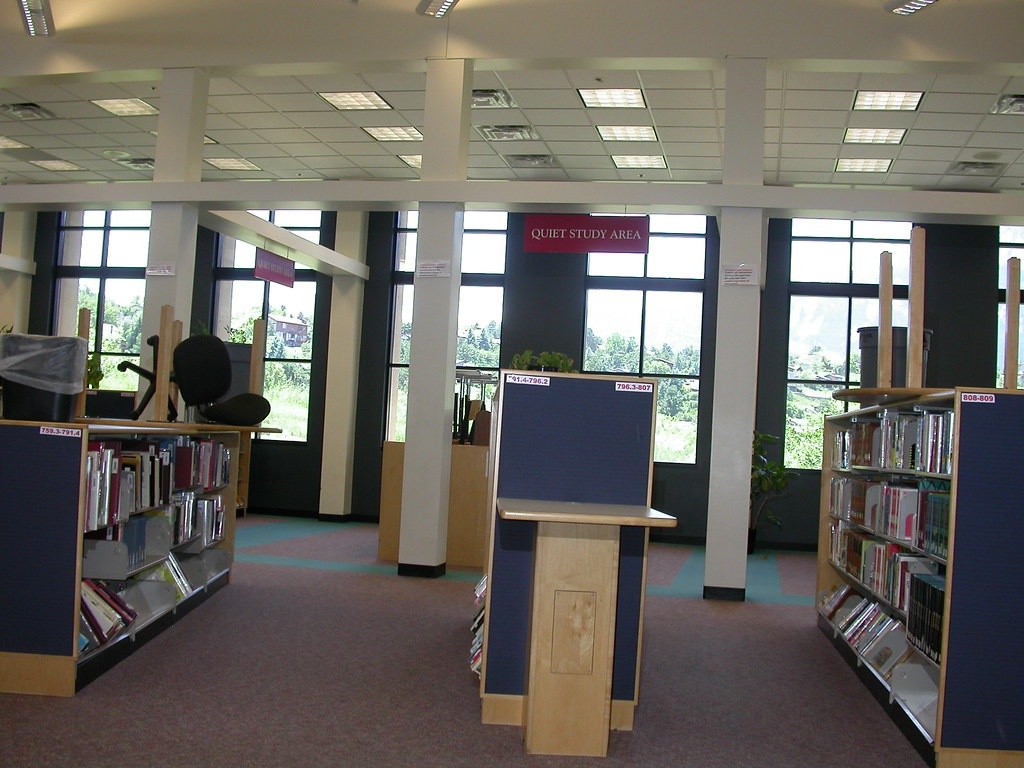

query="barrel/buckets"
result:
[857,326,933,409]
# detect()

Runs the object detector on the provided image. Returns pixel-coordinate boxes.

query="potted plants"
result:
[747,431,799,554]
[510,351,573,372]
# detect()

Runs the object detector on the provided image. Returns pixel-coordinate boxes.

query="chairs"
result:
[117,335,271,426]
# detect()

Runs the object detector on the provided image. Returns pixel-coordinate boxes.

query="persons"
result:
[78,436,231,656]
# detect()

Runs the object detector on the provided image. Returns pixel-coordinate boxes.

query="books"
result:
[818,410,954,683]
[469,572,487,676]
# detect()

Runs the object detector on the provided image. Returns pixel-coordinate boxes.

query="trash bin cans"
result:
[0,333,89,422]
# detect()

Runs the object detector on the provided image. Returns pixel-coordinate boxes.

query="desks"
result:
[832,226,1023,402]
[70,305,282,433]
[496,498,677,757]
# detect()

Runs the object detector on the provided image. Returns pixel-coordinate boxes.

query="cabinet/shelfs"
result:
[0,419,240,697]
[481,368,658,730]
[378,442,483,567]
[236,431,251,517]
[817,387,1024,768]
